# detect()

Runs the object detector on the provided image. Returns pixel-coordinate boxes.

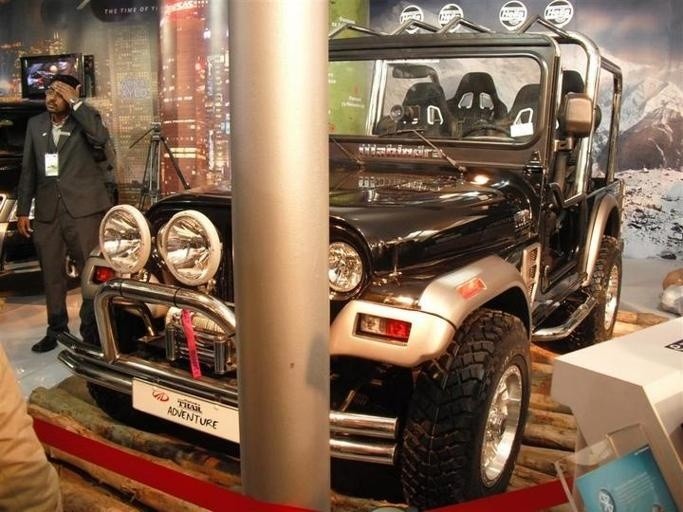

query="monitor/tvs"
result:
[20,52,87,99]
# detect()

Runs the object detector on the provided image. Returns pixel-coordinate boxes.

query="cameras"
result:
[390,105,421,124]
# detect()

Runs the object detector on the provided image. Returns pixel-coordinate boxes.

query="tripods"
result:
[138,133,192,213]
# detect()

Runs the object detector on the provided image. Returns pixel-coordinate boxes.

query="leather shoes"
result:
[32,335,58,352]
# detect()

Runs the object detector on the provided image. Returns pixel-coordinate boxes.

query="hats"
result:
[51,74,79,89]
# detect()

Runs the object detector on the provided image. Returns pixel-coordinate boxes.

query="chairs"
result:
[377,70,601,140]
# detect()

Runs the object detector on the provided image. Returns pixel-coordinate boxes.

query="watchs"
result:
[69,96,81,110]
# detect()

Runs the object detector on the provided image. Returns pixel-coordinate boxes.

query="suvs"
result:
[55,15,627,510]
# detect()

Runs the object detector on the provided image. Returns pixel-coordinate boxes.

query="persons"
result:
[0,345,63,512]
[15,75,117,352]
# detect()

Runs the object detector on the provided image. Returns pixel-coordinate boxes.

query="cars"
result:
[1,100,117,287]
